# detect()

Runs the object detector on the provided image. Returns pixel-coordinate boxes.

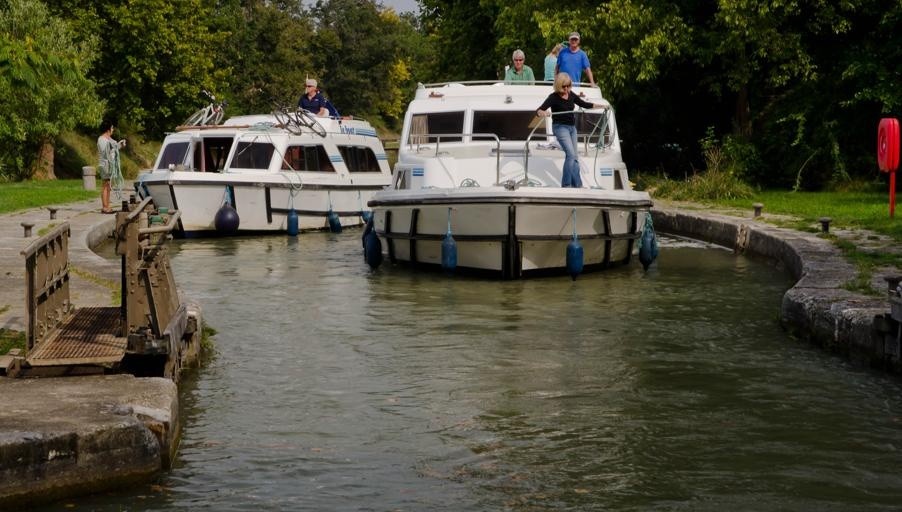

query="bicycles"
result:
[267,97,327,137]
[180,90,227,127]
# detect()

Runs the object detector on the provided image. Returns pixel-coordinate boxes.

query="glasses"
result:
[514,59,524,61]
[305,85,315,88]
[561,84,570,88]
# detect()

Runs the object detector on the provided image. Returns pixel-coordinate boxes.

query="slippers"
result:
[102,207,116,213]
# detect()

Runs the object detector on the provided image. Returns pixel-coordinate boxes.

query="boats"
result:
[361,80,659,280]
[133,113,392,240]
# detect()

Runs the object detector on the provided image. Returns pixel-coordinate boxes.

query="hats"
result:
[569,32,580,40]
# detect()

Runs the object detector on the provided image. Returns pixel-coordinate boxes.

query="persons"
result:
[535,71,610,187]
[503,49,537,85]
[554,31,597,87]
[297,79,326,119]
[542,42,565,82]
[96,119,127,214]
